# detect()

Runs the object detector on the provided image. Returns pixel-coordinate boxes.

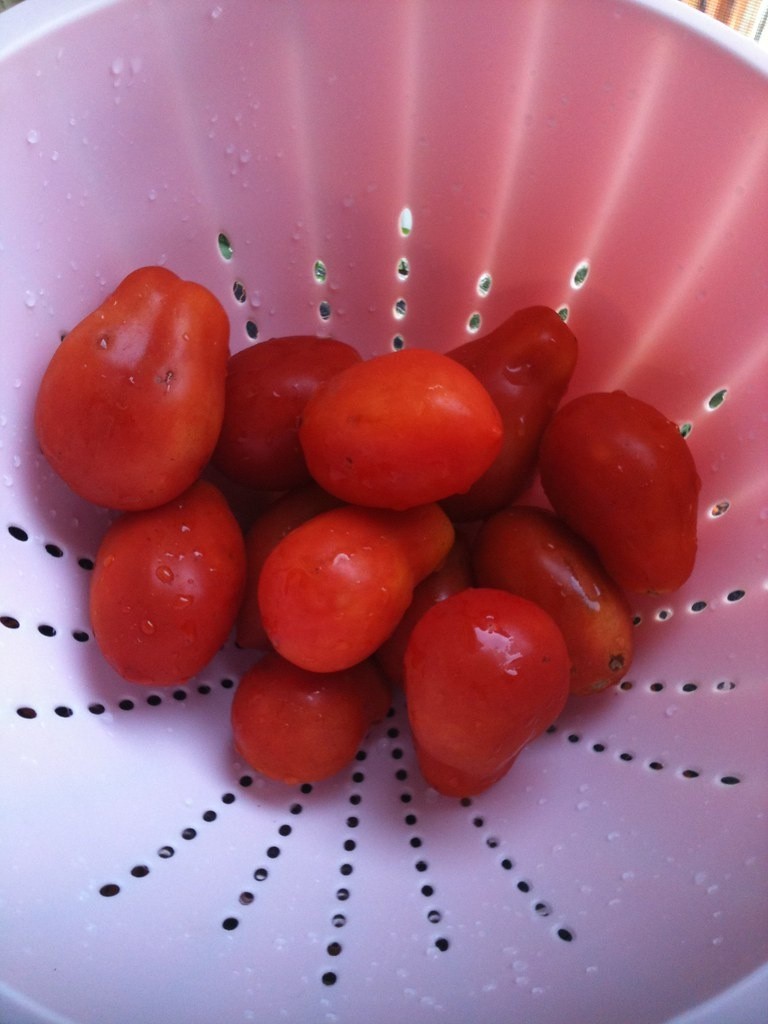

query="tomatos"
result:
[36,267,698,799]
